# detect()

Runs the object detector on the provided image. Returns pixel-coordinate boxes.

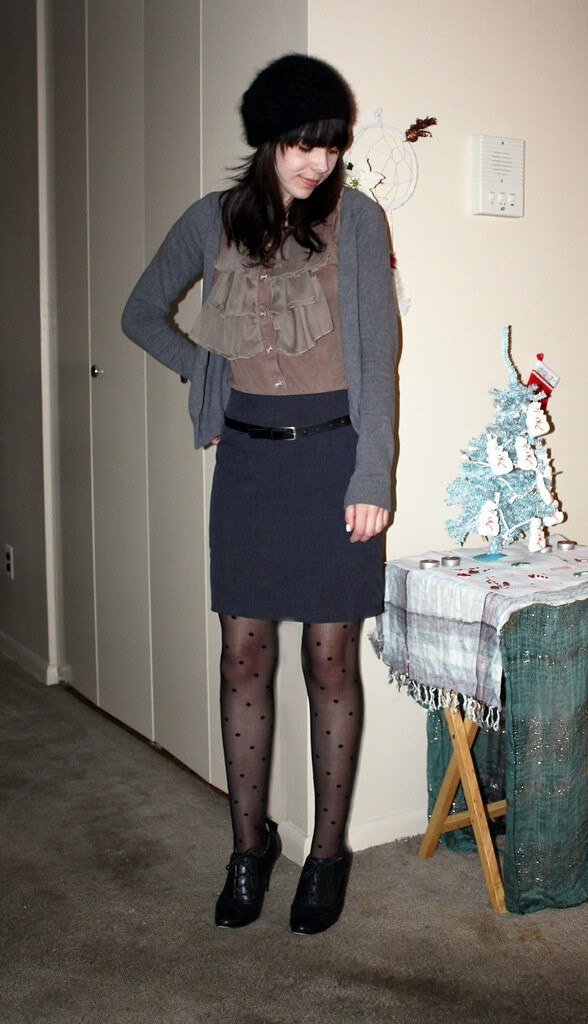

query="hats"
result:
[239,54,356,148]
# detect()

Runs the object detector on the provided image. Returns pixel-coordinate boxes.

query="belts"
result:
[224,413,352,441]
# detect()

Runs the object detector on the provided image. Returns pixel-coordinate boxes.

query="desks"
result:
[369,535,588,914]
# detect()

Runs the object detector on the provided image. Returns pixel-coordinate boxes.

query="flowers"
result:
[345,155,385,203]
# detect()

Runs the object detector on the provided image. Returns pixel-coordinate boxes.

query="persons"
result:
[122,54,401,938]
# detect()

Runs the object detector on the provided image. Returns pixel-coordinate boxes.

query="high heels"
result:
[214,819,282,929]
[289,843,353,934]
